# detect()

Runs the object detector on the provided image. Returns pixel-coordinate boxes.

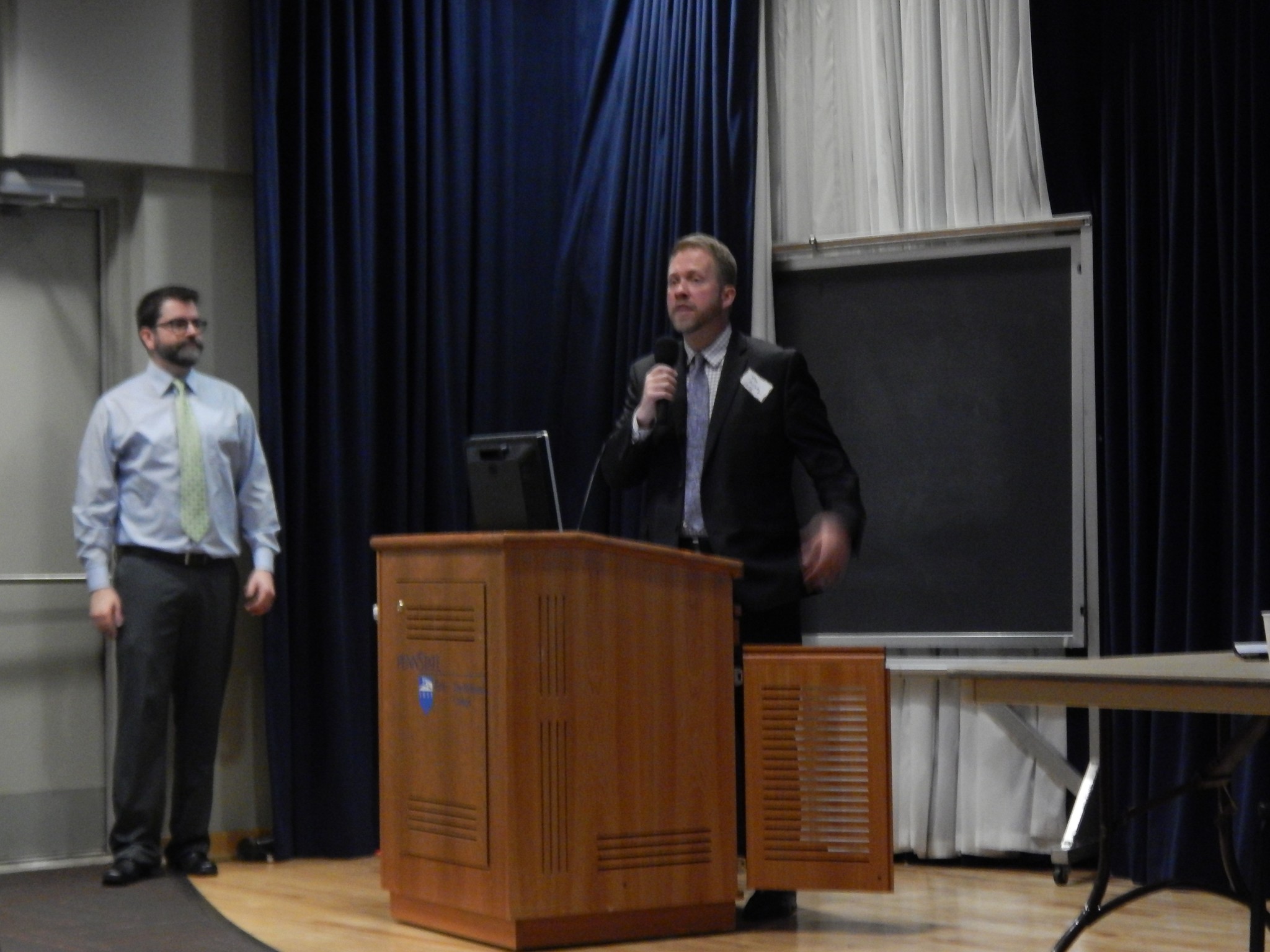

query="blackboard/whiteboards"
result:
[768,212,1097,651]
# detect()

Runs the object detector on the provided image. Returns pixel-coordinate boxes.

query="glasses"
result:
[148,316,210,332]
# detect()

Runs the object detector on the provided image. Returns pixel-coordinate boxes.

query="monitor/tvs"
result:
[465,430,563,533]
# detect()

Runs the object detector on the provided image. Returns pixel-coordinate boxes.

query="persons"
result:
[72,285,284,890]
[597,233,865,935]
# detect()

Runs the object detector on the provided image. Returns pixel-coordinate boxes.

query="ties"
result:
[683,353,711,537]
[170,377,215,540]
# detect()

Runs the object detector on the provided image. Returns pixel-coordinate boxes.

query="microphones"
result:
[654,337,681,413]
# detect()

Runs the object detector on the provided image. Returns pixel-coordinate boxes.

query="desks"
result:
[367,533,896,952]
[952,652,1270,952]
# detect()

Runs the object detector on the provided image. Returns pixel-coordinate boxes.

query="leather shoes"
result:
[104,857,161,886]
[168,851,219,877]
